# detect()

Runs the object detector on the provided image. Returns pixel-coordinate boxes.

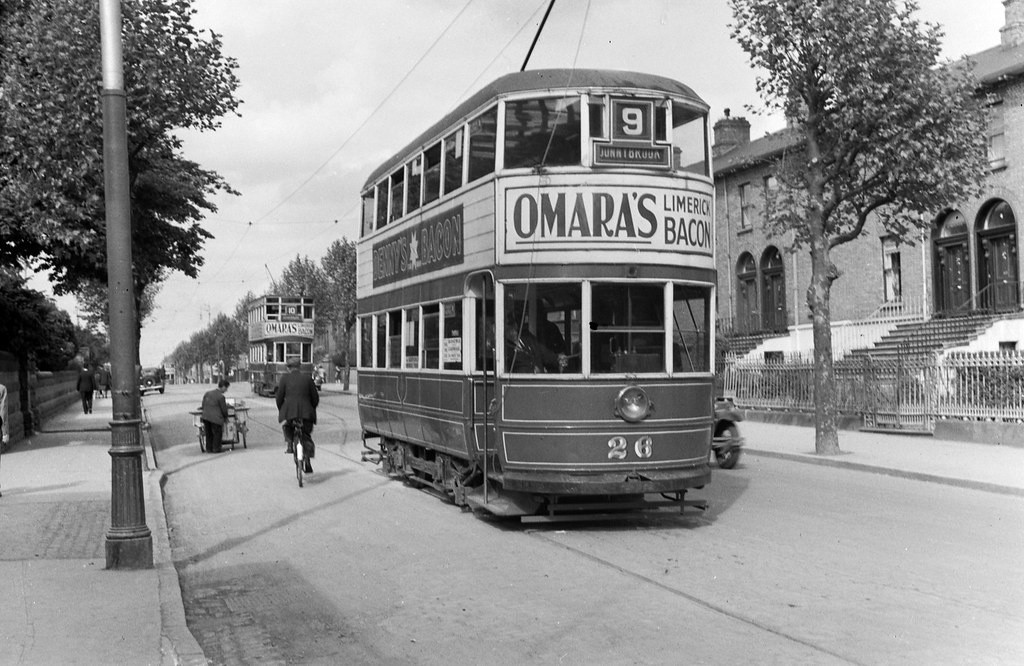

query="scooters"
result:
[713,396,745,468]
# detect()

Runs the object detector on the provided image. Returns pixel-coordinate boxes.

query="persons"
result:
[504,320,555,373]
[276,357,319,473]
[201,381,230,454]
[76,363,111,414]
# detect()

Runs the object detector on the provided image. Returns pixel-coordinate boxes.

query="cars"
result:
[140,366,164,396]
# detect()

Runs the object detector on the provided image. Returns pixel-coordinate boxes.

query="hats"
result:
[285,357,300,367]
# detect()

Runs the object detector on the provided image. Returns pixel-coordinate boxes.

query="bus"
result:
[354,68,720,527]
[244,295,316,397]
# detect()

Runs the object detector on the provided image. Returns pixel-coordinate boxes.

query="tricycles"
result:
[190,399,251,450]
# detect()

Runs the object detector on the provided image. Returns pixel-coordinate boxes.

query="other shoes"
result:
[305,459,312,472]
[288,444,293,452]
[89,406,92,413]
[85,411,88,414]
[208,448,224,453]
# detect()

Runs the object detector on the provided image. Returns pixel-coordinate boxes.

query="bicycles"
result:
[284,419,313,487]
